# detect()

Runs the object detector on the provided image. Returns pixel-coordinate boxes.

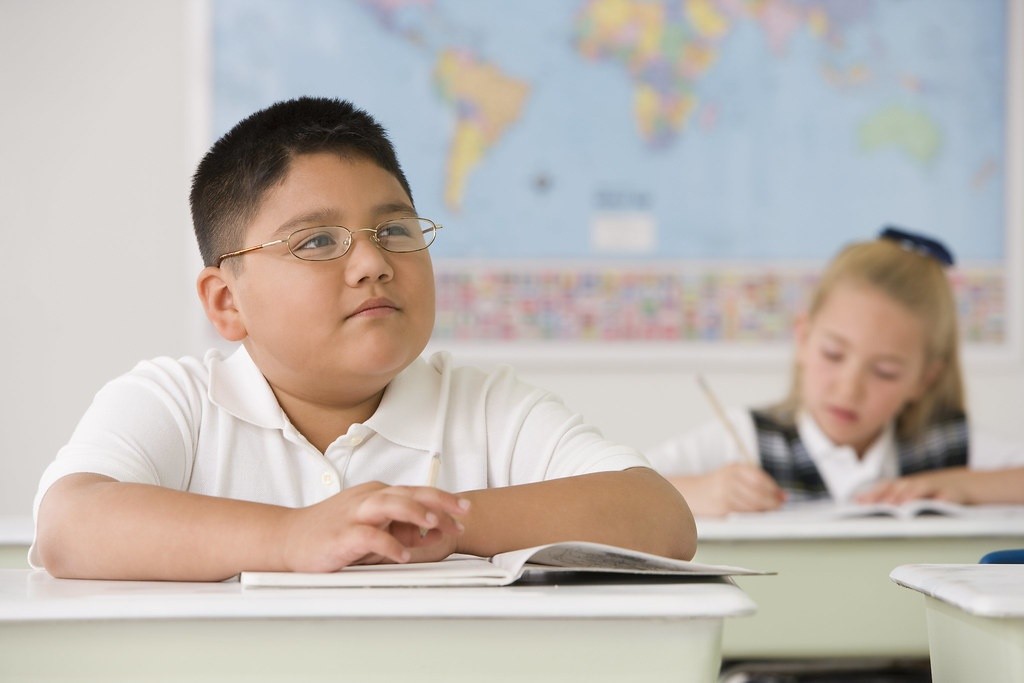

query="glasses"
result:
[214,215,444,269]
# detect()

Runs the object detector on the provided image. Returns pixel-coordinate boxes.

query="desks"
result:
[0,558,753,683]
[887,564,1024,683]
[690,499,1024,661]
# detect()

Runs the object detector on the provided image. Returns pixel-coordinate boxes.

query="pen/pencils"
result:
[690,364,755,468]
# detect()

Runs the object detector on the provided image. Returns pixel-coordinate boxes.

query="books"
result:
[846,500,962,519]
[240,541,777,586]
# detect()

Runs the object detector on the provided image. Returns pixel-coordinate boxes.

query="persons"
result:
[646,227,1024,518]
[26,95,702,577]
